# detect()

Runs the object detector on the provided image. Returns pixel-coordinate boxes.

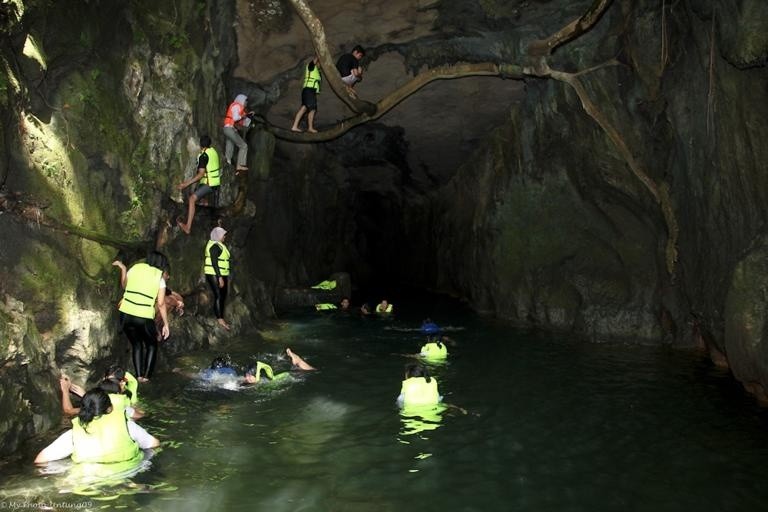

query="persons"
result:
[172,348,319,391]
[176,134,221,235]
[204,226,231,332]
[376,300,393,314]
[360,305,372,316]
[152,261,184,330]
[221,93,256,170]
[33,364,160,469]
[405,333,448,363]
[112,252,170,384]
[397,364,441,410]
[336,44,365,94]
[339,296,351,311]
[291,53,321,133]
[419,317,440,333]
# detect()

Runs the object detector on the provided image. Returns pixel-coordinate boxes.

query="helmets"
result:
[420,322,440,336]
